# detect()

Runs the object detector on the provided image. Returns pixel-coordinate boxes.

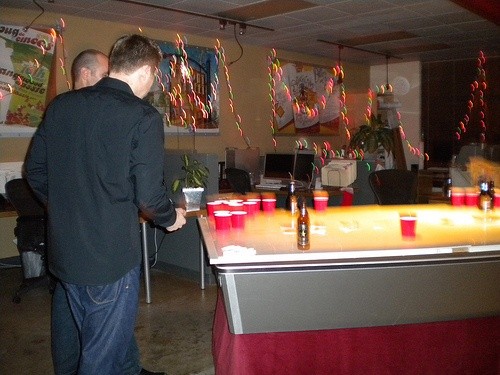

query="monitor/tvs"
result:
[263,152,316,183]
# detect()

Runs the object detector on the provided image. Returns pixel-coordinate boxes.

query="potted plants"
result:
[170,154,210,213]
[352,123,395,161]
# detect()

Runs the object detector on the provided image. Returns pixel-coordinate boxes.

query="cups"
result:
[314,197,329,213]
[207,198,277,234]
[400,216,417,241]
[450,186,500,209]
[340,187,353,207]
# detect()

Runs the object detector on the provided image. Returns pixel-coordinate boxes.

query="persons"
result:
[50,49,109,375]
[22,33,187,374]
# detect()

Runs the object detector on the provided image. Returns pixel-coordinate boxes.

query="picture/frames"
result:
[0,23,60,139]
[145,38,221,137]
[270,57,341,136]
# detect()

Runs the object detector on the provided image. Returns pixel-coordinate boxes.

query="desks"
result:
[136,208,209,303]
[245,180,360,209]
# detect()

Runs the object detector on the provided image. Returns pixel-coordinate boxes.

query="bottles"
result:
[479,180,495,212]
[443,178,452,201]
[297,201,310,250]
[285,182,303,219]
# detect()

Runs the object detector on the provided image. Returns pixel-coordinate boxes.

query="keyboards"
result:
[256,183,287,189]
